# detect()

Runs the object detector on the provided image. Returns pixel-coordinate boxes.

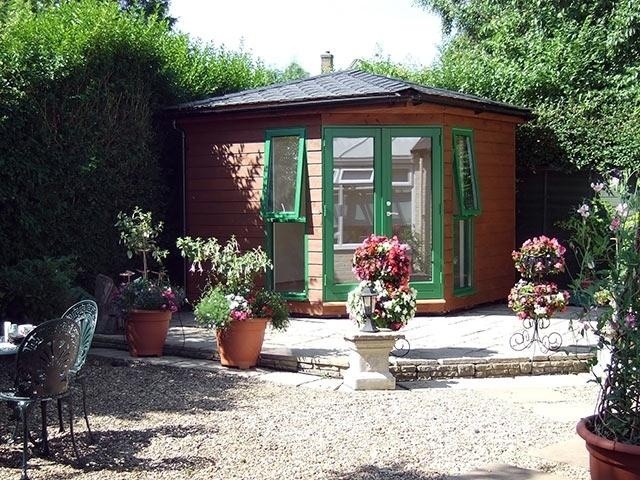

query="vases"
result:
[120,308,173,358]
[214,318,268,369]
[577,412,640,480]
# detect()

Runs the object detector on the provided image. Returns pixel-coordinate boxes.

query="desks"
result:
[0,338,43,356]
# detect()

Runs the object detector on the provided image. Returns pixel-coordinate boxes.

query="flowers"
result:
[508,234,570,320]
[581,178,640,444]
[121,277,187,311]
[193,274,290,332]
[345,233,418,330]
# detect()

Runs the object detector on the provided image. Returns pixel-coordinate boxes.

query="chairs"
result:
[0,318,81,480]
[41,300,98,443]
